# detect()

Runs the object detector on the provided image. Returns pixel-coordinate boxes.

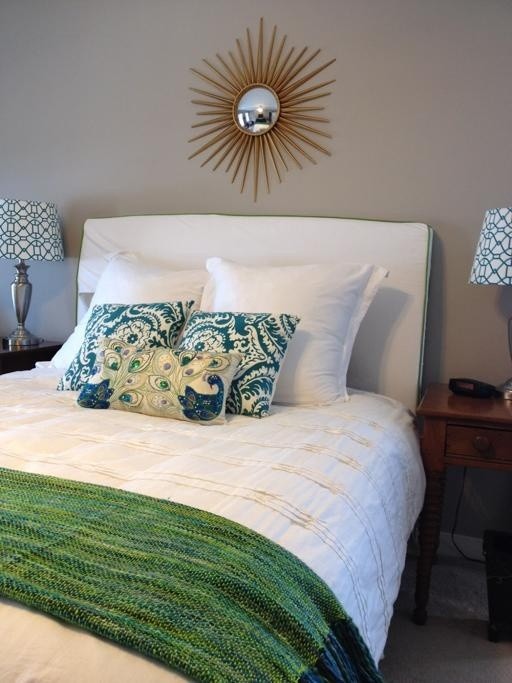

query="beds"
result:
[0,214,433,680]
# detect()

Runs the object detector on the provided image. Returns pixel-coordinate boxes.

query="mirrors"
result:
[186,11,338,205]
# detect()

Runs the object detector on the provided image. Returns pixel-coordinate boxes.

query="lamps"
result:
[466,207,511,402]
[1,198,63,351]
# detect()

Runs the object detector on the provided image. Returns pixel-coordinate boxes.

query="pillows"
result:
[56,301,194,392]
[35,247,208,383]
[201,255,389,407]
[78,335,242,424]
[177,309,300,418]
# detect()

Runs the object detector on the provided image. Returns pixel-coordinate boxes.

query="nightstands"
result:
[408,378,512,627]
[1,337,63,373]
